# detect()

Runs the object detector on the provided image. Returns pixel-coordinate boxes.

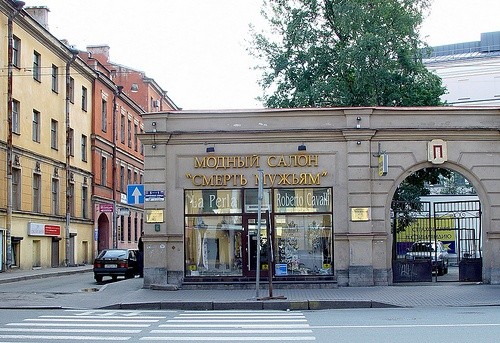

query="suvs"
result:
[405,240,449,276]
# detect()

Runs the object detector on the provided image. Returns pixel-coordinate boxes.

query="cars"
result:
[93,249,138,284]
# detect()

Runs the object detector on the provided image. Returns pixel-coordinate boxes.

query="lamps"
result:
[206,147,215,153]
[298,145,306,151]
